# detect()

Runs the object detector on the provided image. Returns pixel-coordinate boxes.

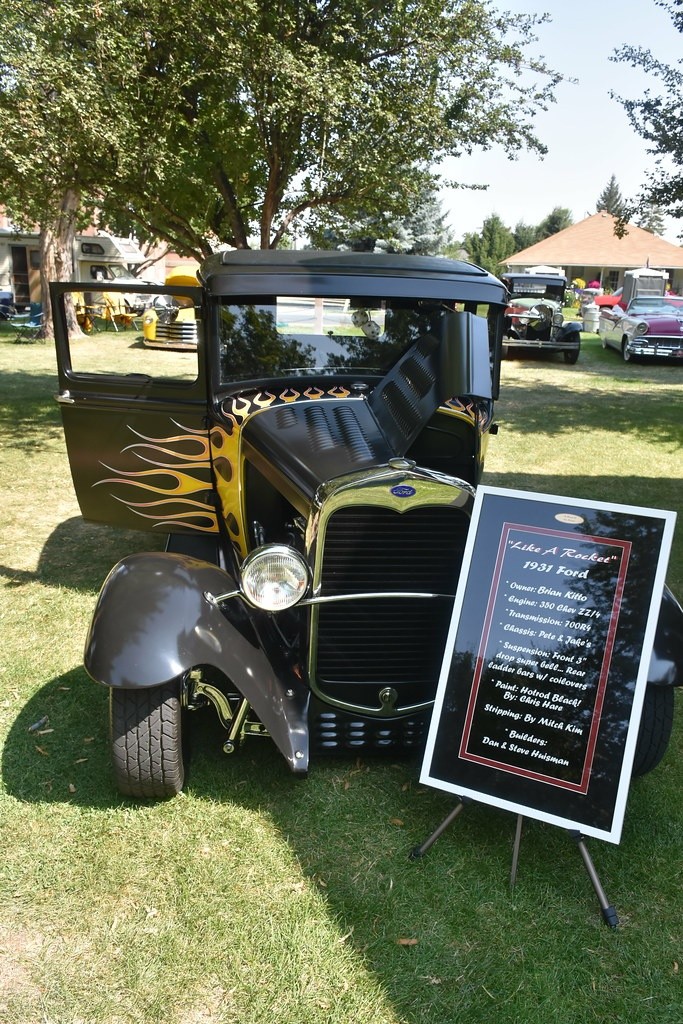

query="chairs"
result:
[0,301,45,343]
[70,292,168,333]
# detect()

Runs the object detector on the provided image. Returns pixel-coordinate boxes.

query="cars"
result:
[45,249,682,802]
[498,269,585,366]
[598,296,682,362]
[142,264,204,351]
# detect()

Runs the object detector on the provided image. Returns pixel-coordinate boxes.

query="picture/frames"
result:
[418,484,679,845]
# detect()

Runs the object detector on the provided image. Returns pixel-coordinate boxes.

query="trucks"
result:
[0,230,167,313]
